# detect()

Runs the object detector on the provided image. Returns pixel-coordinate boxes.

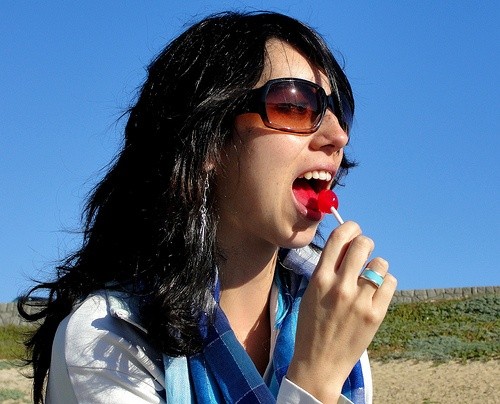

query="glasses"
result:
[240,78,347,133]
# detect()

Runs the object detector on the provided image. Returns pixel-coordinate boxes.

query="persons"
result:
[17,6,398,404]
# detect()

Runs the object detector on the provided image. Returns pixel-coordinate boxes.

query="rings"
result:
[359,267,385,288]
